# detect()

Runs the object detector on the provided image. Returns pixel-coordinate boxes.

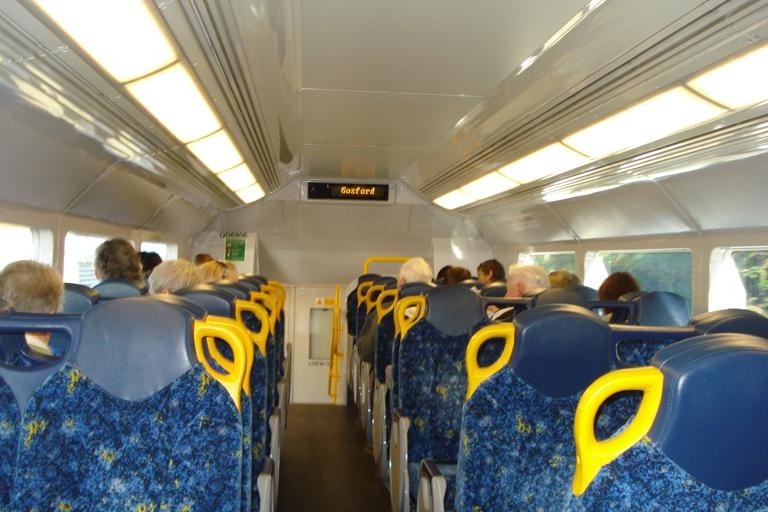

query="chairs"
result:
[340,272,768,512]
[1,270,288,512]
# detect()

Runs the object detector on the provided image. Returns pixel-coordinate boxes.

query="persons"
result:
[0,260,66,359]
[92,234,242,300]
[353,258,643,365]
